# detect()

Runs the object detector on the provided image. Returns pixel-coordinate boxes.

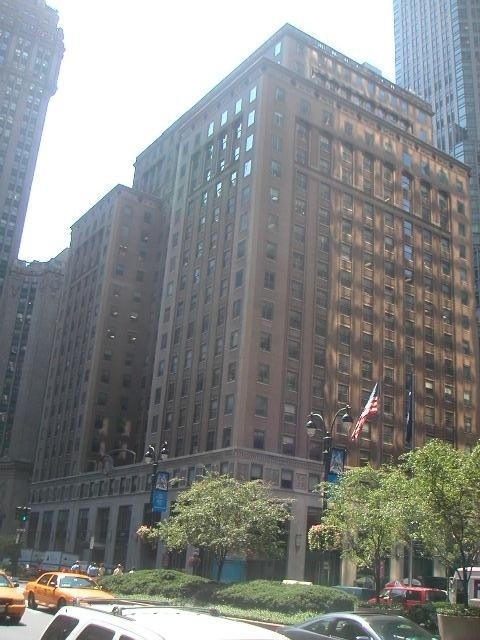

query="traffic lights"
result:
[16,506,31,533]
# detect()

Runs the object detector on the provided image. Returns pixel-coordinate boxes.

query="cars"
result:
[23,571,117,614]
[0,570,26,626]
[277,610,439,640]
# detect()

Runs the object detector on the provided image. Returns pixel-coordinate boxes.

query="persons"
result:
[72,561,135,576]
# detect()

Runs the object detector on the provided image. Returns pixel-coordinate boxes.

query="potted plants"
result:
[435,605,480,640]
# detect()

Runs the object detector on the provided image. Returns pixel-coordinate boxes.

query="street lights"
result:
[144,441,170,568]
[305,403,354,587]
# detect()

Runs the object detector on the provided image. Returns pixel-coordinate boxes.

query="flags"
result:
[404,391,412,450]
[351,383,379,441]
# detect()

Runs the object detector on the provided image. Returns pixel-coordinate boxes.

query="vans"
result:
[367,588,448,613]
[43,600,291,640]
